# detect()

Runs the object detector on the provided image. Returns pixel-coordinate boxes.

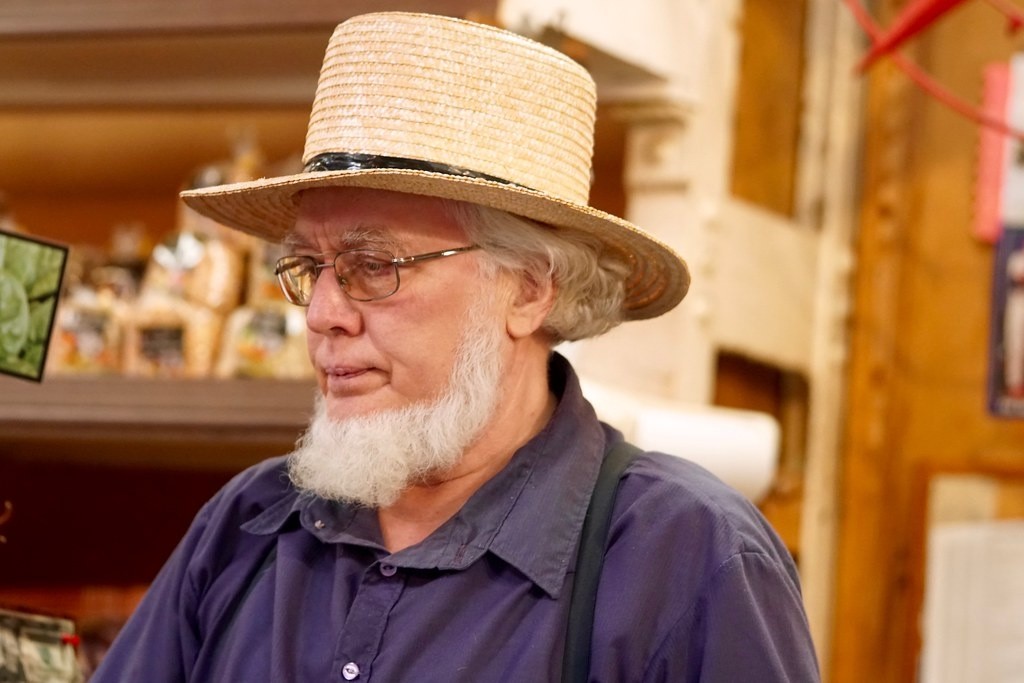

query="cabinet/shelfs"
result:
[0,0,694,478]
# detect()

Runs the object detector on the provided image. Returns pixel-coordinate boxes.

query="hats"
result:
[178,12,691,321]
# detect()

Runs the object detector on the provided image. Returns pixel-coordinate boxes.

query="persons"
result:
[89,9,822,683]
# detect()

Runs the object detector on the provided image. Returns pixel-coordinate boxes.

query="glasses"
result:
[272,244,506,307]
